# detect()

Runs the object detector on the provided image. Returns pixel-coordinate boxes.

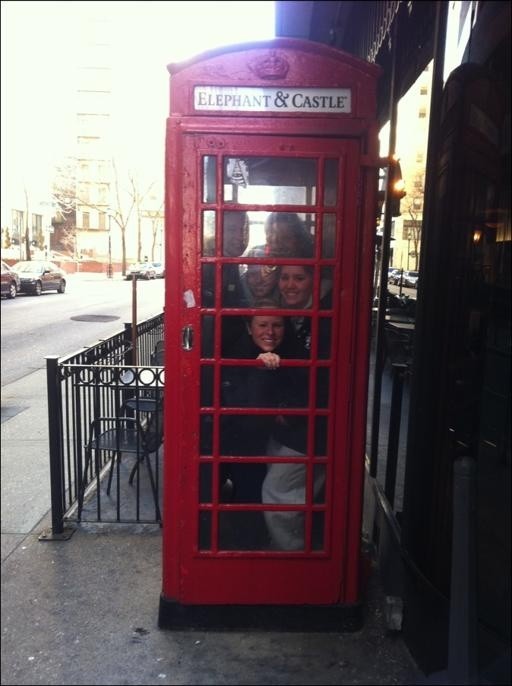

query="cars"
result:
[1,260,66,299]
[126,262,164,280]
[388,266,419,289]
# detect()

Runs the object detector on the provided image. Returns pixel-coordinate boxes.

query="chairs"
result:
[82,414,158,524]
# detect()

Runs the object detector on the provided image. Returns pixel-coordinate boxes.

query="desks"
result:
[107,363,164,487]
